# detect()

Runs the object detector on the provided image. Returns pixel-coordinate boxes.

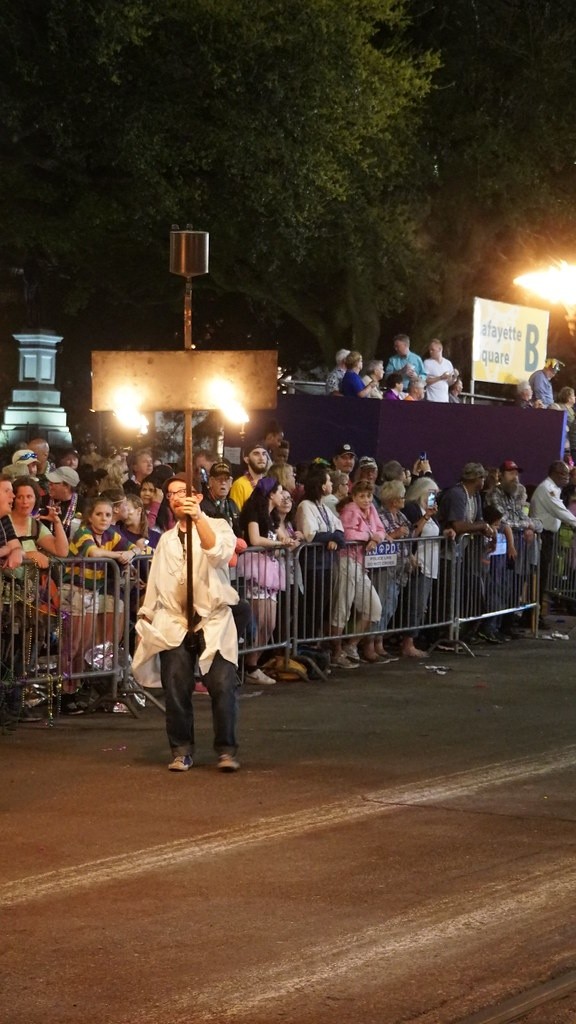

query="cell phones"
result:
[420,452,426,462]
[427,489,436,509]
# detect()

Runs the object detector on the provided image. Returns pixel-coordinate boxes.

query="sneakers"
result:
[217,754,241,770]
[168,754,193,770]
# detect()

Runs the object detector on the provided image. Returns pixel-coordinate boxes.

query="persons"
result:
[423,338,458,403]
[341,351,376,398]
[515,358,576,461]
[325,349,350,396]
[383,372,404,400]
[520,460,576,629]
[384,335,427,392]
[359,360,384,399]
[404,380,425,401]
[449,376,463,403]
[129,472,241,772]
[0,417,543,720]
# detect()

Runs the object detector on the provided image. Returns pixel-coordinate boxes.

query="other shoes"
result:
[7,619,551,721]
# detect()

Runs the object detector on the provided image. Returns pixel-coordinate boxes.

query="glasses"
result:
[15,453,38,461]
[165,489,197,500]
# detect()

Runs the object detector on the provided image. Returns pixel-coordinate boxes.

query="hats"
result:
[359,456,377,469]
[12,450,41,465]
[243,443,263,457]
[500,461,522,473]
[2,464,39,482]
[45,466,80,487]
[334,446,355,457]
[463,463,489,479]
[210,463,231,476]
[163,472,202,493]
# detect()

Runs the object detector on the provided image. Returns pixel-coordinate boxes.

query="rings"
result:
[373,547,374,548]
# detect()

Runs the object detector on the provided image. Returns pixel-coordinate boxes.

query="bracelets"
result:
[423,515,428,521]
[132,549,137,556]
